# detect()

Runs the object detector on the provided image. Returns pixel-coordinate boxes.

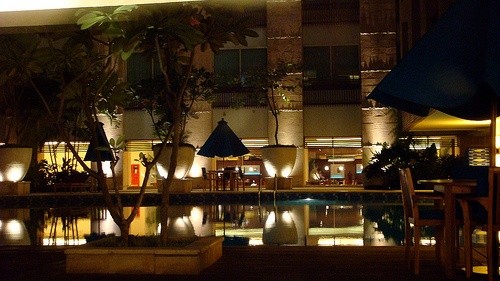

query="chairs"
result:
[455,167,500,281]
[202,167,214,191]
[396,167,461,274]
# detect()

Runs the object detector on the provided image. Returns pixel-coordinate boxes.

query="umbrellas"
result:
[197,117,251,191]
[83,122,114,163]
[366,1,500,119]
[82,220,116,243]
[218,220,249,246]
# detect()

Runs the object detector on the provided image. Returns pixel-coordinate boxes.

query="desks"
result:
[241,172,263,190]
[208,170,239,191]
[417,178,478,281]
